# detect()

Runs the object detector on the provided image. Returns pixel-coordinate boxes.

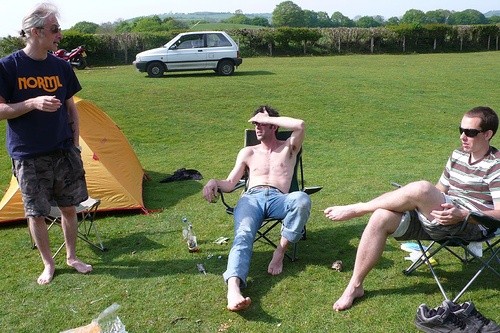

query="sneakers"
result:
[414,303,480,333]
[441,299,500,333]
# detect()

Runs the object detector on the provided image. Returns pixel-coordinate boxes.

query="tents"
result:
[0,96,151,223]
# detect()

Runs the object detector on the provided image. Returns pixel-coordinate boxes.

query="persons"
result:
[0,1,93,285]
[202,106,311,311]
[324,107,500,311]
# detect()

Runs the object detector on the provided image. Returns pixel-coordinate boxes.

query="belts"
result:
[249,185,285,194]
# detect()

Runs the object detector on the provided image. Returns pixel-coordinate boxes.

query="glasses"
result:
[34,27,61,33]
[459,127,484,137]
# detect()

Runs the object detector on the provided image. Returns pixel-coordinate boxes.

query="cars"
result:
[132,30,244,78]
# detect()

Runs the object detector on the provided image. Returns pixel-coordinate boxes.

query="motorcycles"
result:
[49,44,87,71]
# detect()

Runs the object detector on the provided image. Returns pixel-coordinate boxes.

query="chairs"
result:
[22,196,106,259]
[402,212,500,304]
[212,129,324,262]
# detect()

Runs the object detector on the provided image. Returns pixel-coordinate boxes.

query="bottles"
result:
[182,218,190,242]
[187,225,198,253]
